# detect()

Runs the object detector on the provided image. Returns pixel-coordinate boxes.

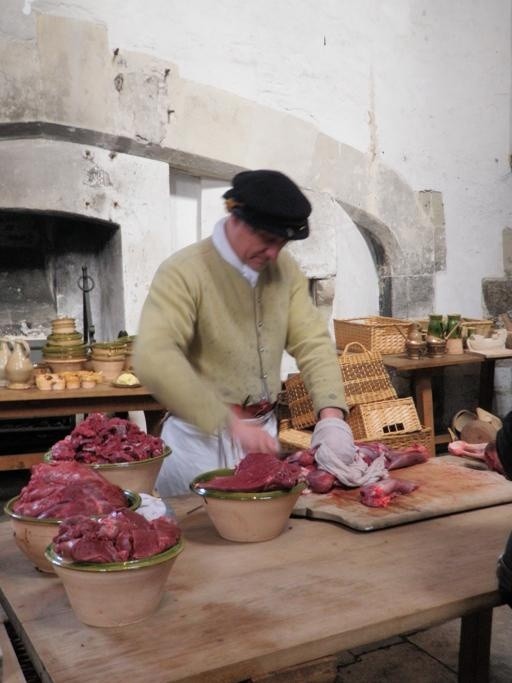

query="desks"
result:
[337,348,512,455]
[2,454,512,681]
[2,382,182,507]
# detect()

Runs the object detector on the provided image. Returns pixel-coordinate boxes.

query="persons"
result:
[129,170,357,497]
[495,409,512,611]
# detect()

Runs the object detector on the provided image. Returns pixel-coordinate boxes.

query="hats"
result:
[219,169,312,241]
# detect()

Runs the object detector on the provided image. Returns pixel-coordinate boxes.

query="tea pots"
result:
[0,336,34,390]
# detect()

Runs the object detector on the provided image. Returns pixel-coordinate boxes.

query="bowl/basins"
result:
[42,530,186,626]
[4,488,142,578]
[189,469,309,542]
[29,317,142,390]
[38,441,172,500]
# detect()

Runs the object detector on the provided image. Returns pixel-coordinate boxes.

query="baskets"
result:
[416,314,493,343]
[332,314,411,356]
[275,342,436,462]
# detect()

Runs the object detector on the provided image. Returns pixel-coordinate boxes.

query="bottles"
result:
[402,313,465,361]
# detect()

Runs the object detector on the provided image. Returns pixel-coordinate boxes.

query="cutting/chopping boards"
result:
[286,452,512,532]
